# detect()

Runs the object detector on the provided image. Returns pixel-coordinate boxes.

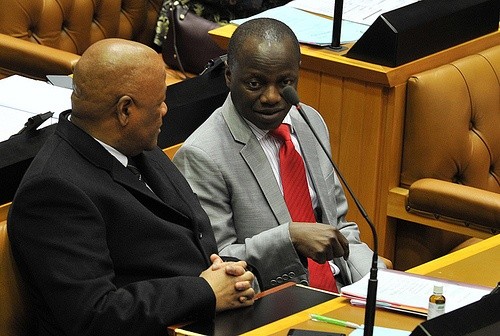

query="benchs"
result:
[0,0,199,87]
[0,142,185,336]
[383,44,500,271]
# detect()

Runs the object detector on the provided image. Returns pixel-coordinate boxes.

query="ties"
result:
[269,123,338,294]
[128,163,145,182]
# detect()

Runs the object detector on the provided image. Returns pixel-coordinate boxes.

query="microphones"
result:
[282,86,379,336]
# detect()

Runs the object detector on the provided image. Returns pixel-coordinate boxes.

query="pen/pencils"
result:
[310,314,365,329]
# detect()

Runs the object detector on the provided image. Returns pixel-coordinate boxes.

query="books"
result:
[230,6,370,46]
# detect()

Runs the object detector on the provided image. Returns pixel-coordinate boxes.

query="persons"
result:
[172,17,387,292]
[6,38,265,336]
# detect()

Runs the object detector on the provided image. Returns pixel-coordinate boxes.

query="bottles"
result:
[427,284,447,320]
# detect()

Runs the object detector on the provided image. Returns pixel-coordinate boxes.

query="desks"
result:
[206,11,500,263]
[167,234,500,336]
